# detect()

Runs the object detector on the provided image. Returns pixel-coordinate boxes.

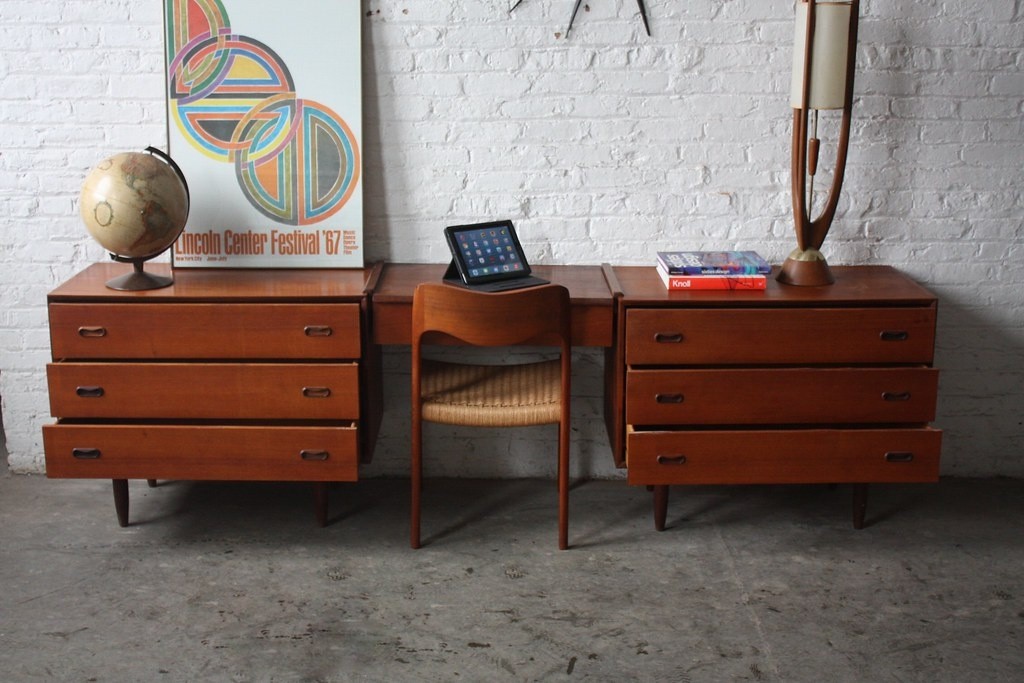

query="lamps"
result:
[775,0,859,287]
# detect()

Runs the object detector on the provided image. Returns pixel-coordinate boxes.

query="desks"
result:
[362,262,623,348]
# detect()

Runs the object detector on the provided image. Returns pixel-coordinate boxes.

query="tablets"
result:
[444,219,532,285]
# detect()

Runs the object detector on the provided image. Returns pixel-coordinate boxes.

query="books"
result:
[657,250,772,291]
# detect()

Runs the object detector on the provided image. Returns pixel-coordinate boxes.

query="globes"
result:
[78,146,189,293]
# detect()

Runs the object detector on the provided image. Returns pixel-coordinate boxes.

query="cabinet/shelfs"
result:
[602,264,943,531]
[41,258,384,526]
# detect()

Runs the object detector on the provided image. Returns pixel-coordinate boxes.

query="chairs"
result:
[411,282,572,549]
